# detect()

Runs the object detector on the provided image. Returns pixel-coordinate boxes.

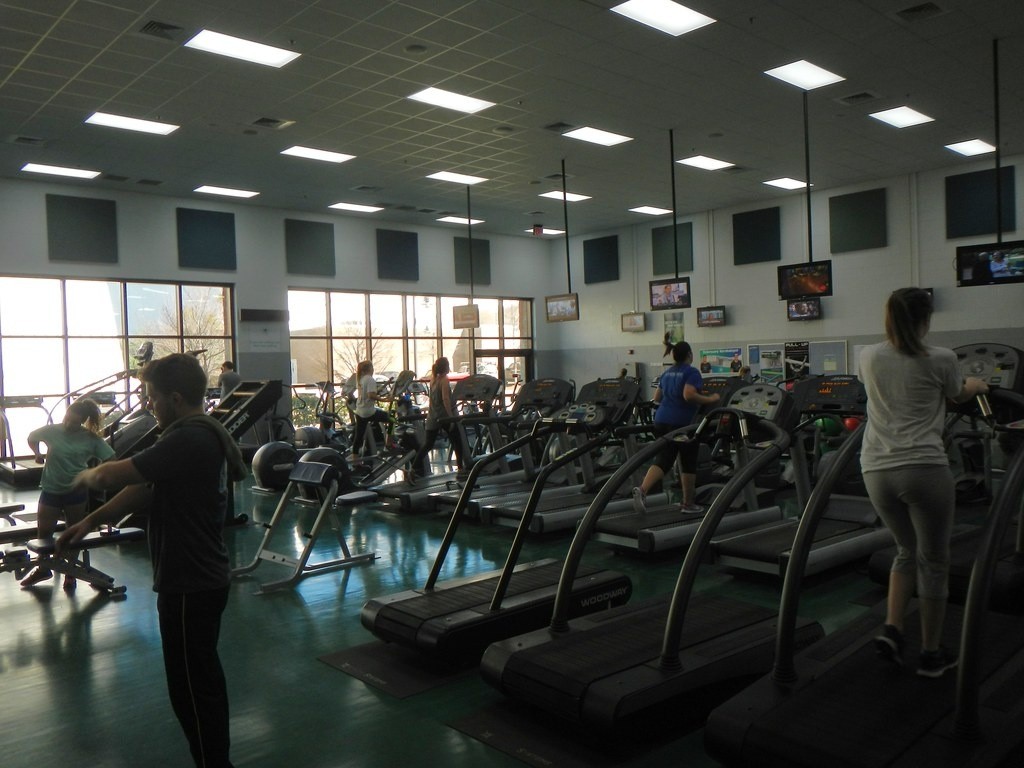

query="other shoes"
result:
[352,464,371,472]
[384,446,395,452]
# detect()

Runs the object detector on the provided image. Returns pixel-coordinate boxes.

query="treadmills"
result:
[477,384,826,736]
[367,374,1024,601]
[704,342,1024,767]
[360,401,633,653]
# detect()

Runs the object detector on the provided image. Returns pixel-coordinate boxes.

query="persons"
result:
[973,252,1012,278]
[551,301,575,317]
[656,285,715,321]
[20,399,117,591]
[632,331,721,514]
[350,357,471,487]
[702,353,742,374]
[858,287,990,679]
[738,366,752,384]
[47,353,243,768]
[790,301,818,316]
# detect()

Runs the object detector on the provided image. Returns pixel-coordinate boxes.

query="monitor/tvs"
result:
[650,277,691,311]
[787,298,821,321]
[621,312,647,332]
[956,240,1024,287]
[777,260,833,301]
[696,305,726,327]
[545,293,580,323]
[453,304,479,329]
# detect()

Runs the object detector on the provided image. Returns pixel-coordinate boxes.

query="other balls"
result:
[843,417,860,431]
[815,417,843,437]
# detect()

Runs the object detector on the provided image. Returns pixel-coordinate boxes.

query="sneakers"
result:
[21,569,53,586]
[64,574,76,589]
[456,471,469,480]
[632,487,647,515]
[917,645,960,677]
[875,624,907,669]
[681,504,704,513]
[407,469,416,485]
